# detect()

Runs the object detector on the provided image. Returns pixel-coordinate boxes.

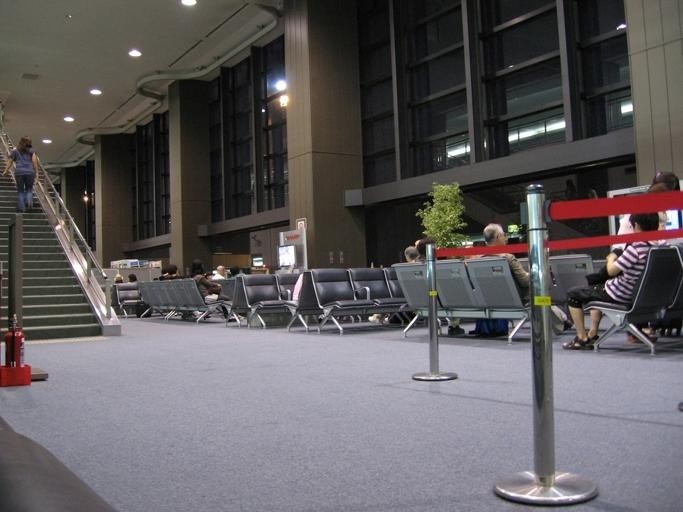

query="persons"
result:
[127,273,137,282]
[112,274,123,284]
[188,257,239,322]
[229,264,246,278]
[365,223,573,335]
[1,136,37,214]
[559,211,659,351]
[212,265,224,279]
[158,263,184,280]
[609,171,682,340]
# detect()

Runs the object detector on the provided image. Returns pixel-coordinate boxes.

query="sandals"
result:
[562,335,599,350]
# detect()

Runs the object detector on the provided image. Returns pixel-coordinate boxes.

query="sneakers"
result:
[448,325,464,335]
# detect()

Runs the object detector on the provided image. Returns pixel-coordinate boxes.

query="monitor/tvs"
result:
[252,257,264,266]
[664,210,681,230]
[277,244,297,268]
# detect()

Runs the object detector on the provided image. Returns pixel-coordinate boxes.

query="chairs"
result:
[113,245,683,356]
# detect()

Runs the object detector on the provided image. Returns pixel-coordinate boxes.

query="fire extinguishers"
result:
[5,314,24,367]
